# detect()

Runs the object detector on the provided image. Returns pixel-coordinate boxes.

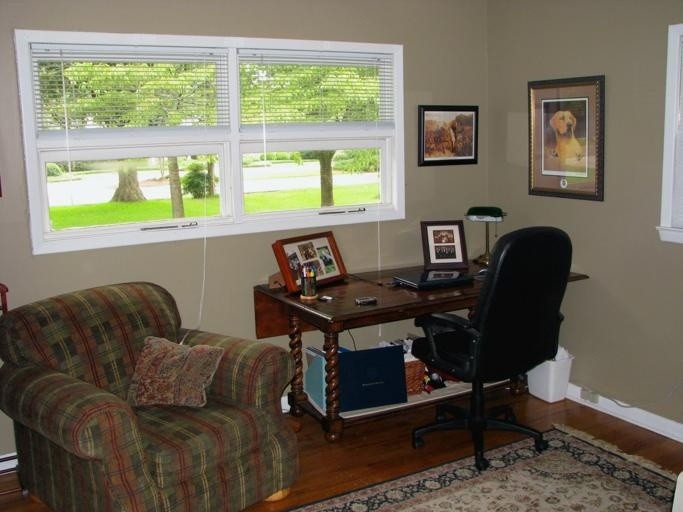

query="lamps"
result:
[464,203,505,274]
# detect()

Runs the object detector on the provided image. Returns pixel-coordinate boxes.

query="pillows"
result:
[125,335,225,413]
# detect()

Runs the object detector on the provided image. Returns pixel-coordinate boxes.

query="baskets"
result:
[404,360,424,396]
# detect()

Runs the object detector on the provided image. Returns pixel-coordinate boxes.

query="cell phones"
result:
[356,297,377,305]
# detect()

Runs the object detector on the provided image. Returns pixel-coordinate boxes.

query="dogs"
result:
[548,110,588,165]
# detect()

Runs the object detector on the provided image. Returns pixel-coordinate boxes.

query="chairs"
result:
[1,278,302,511]
[405,225,577,475]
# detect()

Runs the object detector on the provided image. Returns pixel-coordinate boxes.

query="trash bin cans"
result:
[527,352,575,404]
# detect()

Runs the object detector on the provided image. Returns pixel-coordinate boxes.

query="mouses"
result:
[477,269,489,274]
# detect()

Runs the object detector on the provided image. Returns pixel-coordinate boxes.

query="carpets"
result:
[289,422,676,510]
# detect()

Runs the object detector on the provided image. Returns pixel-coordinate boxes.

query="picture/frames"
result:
[269,229,348,293]
[524,75,608,205]
[418,219,471,273]
[415,101,481,168]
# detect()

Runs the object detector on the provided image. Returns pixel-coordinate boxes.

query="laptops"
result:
[394,268,474,291]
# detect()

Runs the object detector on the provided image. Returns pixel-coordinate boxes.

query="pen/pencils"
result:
[298,262,317,296]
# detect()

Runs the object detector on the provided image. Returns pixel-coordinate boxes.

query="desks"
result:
[252,254,589,444]
